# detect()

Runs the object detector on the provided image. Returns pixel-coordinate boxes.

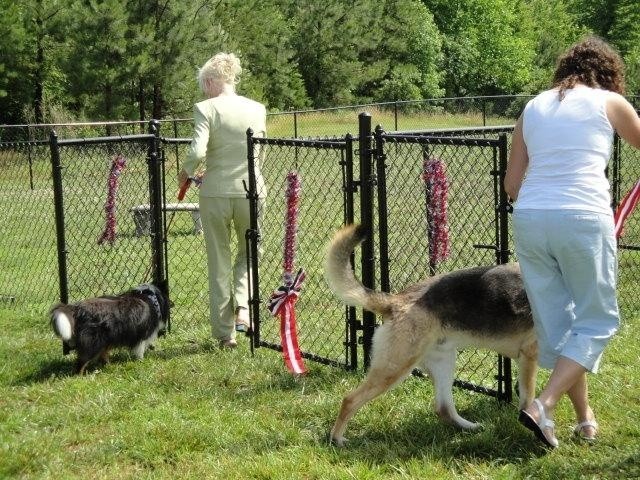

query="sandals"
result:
[236,319,262,336]
[575,420,597,445]
[518,399,558,450]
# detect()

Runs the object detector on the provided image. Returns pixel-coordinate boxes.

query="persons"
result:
[502,37,640,450]
[177,51,266,349]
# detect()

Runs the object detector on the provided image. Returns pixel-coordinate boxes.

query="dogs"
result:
[320,221,540,449]
[48,283,175,376]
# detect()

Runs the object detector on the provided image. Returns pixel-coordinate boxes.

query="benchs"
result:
[128,203,204,238]
